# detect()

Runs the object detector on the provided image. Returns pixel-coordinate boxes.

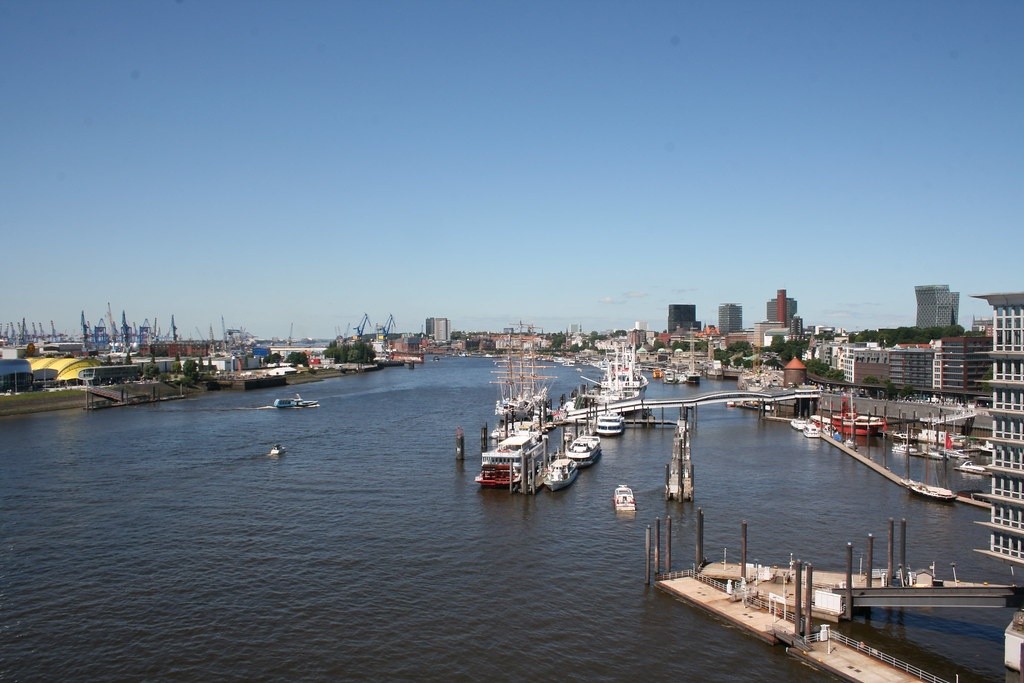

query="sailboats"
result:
[489,319,558,423]
[549,343,645,426]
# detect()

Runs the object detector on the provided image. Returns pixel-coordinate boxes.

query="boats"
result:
[898,479,958,504]
[594,406,626,435]
[471,424,546,486]
[653,324,702,386]
[942,448,968,460]
[274,394,319,408]
[810,397,887,445]
[432,356,439,362]
[271,444,285,454]
[544,459,578,493]
[921,449,946,461]
[614,483,635,511]
[788,414,843,442]
[483,417,557,448]
[565,436,603,468]
[889,442,917,455]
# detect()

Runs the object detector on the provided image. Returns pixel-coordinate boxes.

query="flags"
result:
[946,433,953,450]
[830,398,856,419]
[882,420,887,433]
